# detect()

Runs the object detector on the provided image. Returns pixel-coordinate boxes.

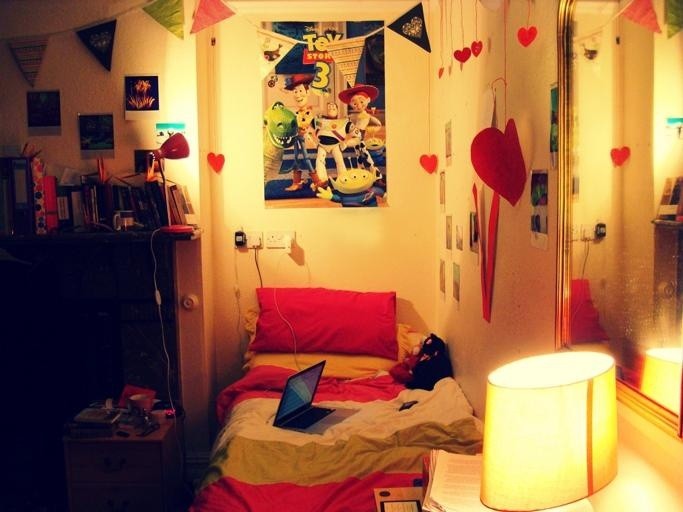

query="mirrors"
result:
[550,0,682,442]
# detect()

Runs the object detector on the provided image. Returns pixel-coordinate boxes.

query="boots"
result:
[284,170,303,191]
[308,170,328,191]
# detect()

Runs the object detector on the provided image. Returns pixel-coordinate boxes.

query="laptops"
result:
[273,359,336,432]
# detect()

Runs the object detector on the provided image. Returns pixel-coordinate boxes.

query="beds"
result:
[190,315,483,512]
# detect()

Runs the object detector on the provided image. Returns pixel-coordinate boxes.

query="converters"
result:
[284,234,295,255]
[235,232,246,246]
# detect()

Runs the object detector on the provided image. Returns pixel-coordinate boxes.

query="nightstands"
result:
[63,405,185,512]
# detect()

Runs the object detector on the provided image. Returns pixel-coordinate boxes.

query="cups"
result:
[113,210,134,232]
[129,393,150,413]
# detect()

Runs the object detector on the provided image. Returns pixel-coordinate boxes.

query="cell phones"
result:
[399,400,417,411]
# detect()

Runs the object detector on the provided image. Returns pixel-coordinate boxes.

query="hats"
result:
[338,83,379,104]
[284,74,314,90]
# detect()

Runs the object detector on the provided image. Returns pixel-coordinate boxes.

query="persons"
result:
[283,73,328,193]
[309,102,354,180]
[338,84,382,180]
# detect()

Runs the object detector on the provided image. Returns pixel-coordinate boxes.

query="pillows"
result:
[250,286,398,363]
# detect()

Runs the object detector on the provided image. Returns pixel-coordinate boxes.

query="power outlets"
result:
[233,230,245,249]
[264,230,296,249]
[595,222,608,240]
[246,232,262,249]
[580,223,593,241]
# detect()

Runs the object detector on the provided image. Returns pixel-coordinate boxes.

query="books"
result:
[31,159,194,235]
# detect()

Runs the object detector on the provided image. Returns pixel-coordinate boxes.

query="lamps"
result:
[481,348,617,512]
[641,346,682,419]
[150,132,195,233]
